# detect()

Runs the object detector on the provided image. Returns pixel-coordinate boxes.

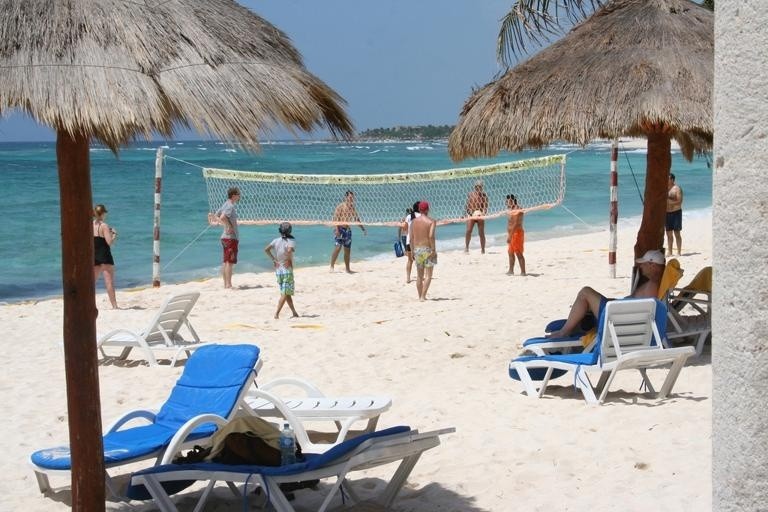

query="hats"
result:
[419,202,428,211]
[279,222,292,236]
[635,249,665,265]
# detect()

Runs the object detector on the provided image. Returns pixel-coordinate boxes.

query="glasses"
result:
[235,193,240,195]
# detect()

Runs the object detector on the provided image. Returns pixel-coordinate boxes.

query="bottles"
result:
[280,424,296,464]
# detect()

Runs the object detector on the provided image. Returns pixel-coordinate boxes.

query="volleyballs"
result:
[472,210,483,216]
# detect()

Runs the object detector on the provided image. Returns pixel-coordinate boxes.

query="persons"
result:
[462,179,487,254]
[525,248,667,357]
[330,190,366,274]
[398,201,437,302]
[264,222,299,320]
[92,205,119,309]
[505,194,526,276]
[665,174,682,257]
[214,187,241,289]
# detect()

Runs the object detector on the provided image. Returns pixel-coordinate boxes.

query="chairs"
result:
[98,292,217,367]
[234,377,394,441]
[131,426,455,512]
[31,345,263,501]
[509,259,712,406]
